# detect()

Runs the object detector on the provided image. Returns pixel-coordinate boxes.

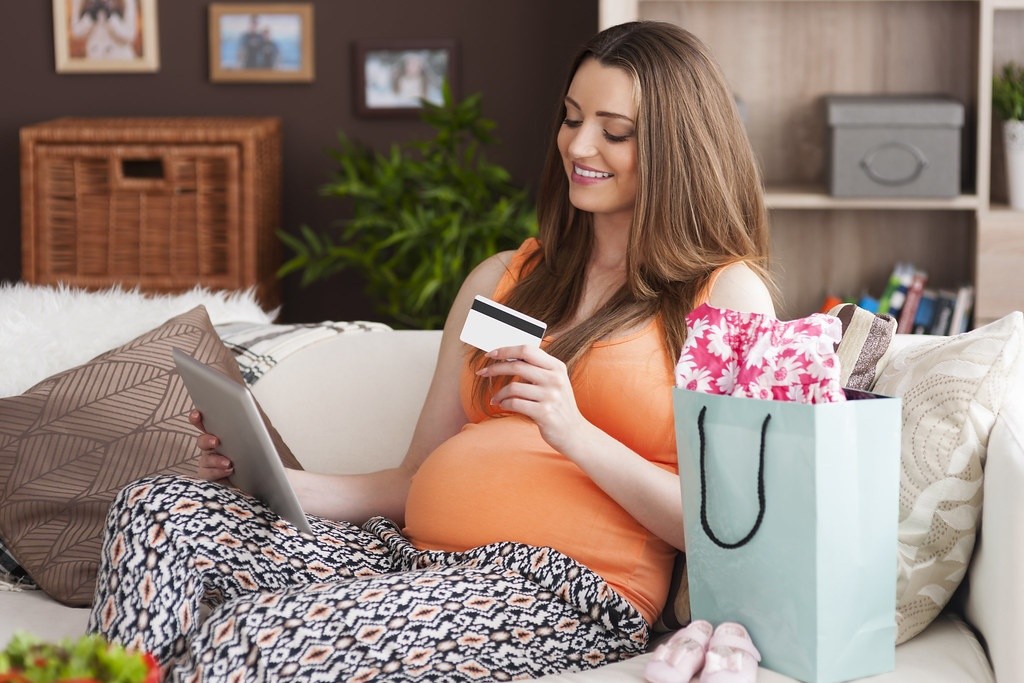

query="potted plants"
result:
[993,60,1024,212]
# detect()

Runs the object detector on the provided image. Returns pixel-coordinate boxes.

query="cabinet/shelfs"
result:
[598,0,1024,329]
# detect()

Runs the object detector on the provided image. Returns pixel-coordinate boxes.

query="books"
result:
[820,263,973,336]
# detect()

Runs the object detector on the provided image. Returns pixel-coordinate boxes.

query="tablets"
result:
[172,346,314,535]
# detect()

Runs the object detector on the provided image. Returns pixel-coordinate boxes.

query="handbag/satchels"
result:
[672,380,902,683]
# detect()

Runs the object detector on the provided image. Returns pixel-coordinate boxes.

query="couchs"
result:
[0,282,1024,683]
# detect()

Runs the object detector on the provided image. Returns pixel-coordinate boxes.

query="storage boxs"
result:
[824,93,965,201]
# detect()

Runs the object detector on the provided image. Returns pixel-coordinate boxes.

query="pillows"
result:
[0,305,304,610]
[886,309,1024,648]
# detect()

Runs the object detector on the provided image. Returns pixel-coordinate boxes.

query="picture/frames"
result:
[51,0,461,120]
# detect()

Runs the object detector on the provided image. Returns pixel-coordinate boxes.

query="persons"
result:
[237,14,278,70]
[392,54,428,98]
[72,0,138,59]
[86,21,776,683]
